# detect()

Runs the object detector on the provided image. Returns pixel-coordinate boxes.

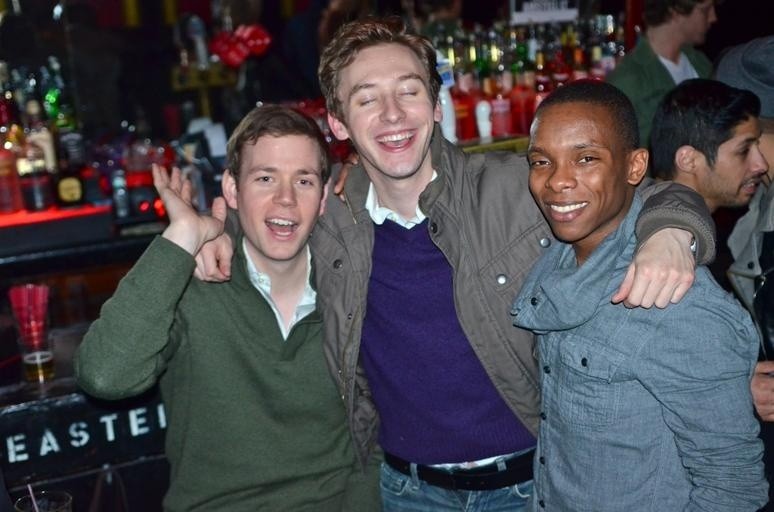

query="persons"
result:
[188,15,719,512]
[600,0,773,512]
[73,103,383,511]
[332,75,772,512]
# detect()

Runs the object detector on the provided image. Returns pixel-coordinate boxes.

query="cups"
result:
[16,307,57,383]
[13,491,73,512]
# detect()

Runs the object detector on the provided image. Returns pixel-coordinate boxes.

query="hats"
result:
[716,37,773,121]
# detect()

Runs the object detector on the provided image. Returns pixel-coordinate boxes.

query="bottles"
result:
[0,54,85,222]
[417,14,628,151]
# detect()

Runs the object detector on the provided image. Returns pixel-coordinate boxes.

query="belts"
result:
[383,448,533,492]
